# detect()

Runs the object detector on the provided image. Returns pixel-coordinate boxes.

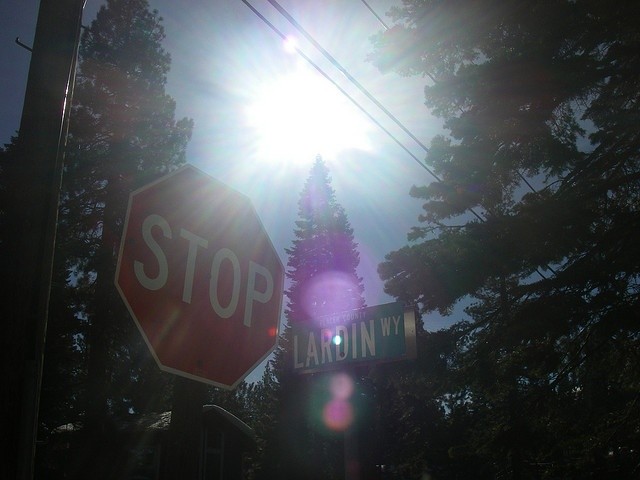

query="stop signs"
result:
[114,162,286,392]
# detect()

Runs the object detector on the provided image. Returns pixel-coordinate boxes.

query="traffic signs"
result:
[292,300,405,374]
